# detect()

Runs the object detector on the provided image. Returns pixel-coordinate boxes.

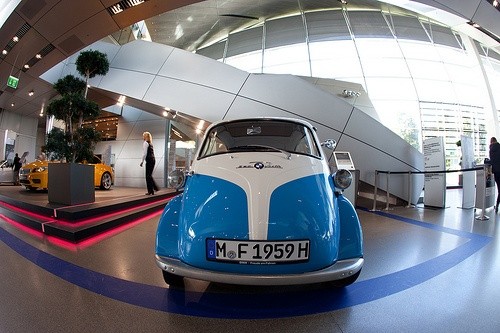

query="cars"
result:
[156,116,364,292]
[0,152,30,183]
[18,150,115,193]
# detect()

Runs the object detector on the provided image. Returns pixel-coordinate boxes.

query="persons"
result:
[484,137,500,214]
[12,153,22,186]
[139,132,160,195]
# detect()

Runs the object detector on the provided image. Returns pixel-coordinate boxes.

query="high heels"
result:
[145,191,154,195]
[155,188,159,191]
